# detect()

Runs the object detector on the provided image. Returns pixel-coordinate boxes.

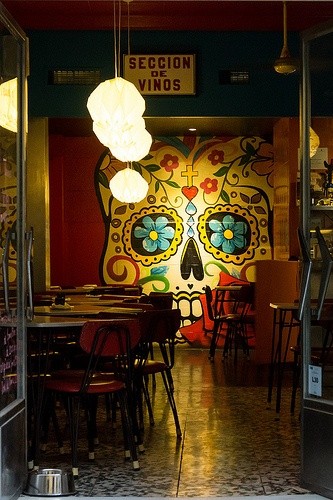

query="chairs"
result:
[291,297,333,420]
[204,282,255,363]
[0,284,182,480]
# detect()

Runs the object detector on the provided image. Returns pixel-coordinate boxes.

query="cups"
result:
[55,293,65,306]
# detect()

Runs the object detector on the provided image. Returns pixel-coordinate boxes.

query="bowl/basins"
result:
[21,469,78,496]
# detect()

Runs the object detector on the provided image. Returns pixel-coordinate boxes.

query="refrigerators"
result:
[0,5,33,500]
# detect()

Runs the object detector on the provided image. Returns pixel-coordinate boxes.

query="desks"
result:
[42,297,124,305]
[9,306,143,315]
[214,284,250,360]
[0,315,131,369]
[312,206,333,230]
[264,302,333,421]
[49,288,139,296]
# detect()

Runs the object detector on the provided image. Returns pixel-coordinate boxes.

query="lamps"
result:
[272,0,297,75]
[86,0,154,205]
